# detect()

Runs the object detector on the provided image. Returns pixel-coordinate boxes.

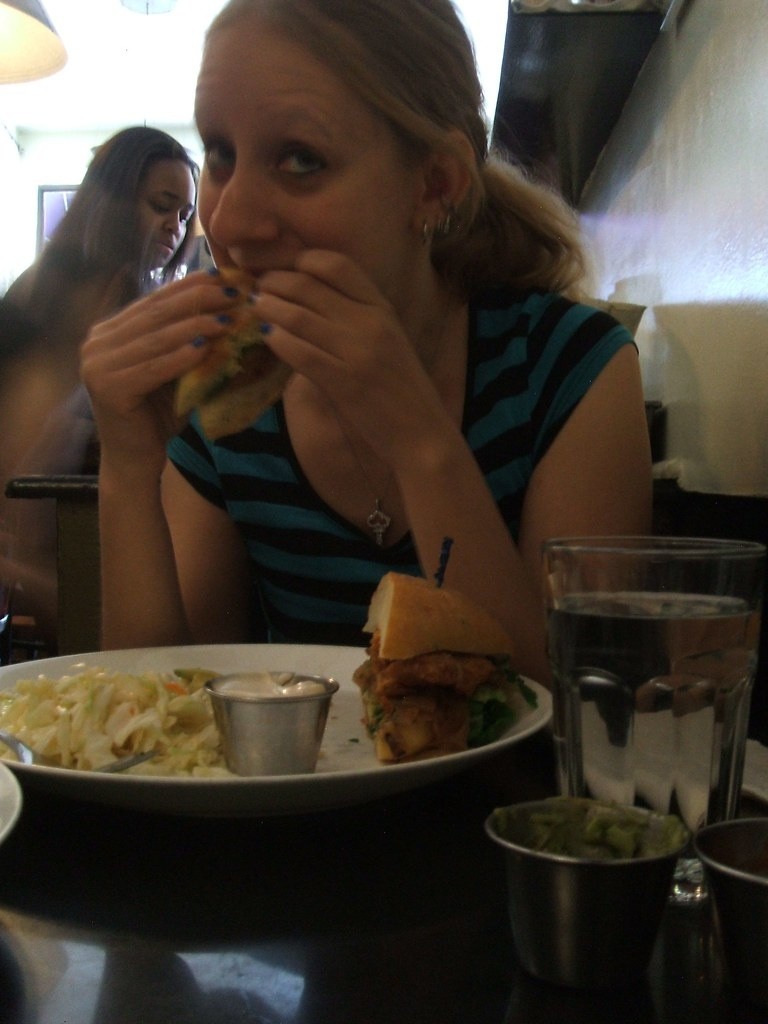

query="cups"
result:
[484,795,690,993]
[654,301,767,496]
[540,535,768,900]
[205,671,339,777]
[694,819,768,983]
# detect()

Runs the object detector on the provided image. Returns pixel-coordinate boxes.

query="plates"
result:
[0,644,552,815]
[0,762,22,843]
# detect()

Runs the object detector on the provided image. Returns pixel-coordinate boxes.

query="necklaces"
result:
[330,287,455,544]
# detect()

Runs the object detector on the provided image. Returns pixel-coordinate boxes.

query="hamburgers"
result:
[352,571,525,765]
[175,267,296,439]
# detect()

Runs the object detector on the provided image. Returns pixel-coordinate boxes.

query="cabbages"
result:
[0,657,230,778]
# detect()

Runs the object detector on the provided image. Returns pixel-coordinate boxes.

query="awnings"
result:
[487,1,673,214]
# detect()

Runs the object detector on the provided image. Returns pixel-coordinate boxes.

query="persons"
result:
[0,125,201,642]
[77,1,655,695]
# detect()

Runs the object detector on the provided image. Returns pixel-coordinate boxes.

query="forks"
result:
[0,723,158,772]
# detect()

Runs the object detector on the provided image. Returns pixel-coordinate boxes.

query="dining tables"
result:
[0,633,768,1024]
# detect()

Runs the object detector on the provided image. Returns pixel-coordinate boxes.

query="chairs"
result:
[4,471,106,658]
